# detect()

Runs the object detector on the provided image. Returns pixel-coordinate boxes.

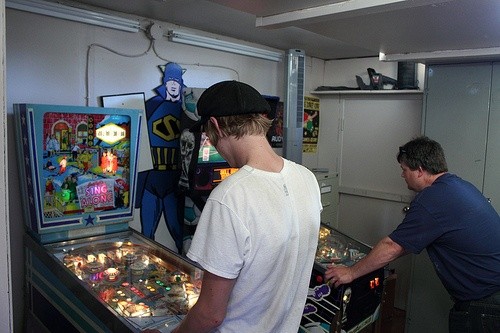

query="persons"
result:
[325,137,500,333]
[140,81,322,333]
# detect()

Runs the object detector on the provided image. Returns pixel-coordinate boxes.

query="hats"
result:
[196,80,271,121]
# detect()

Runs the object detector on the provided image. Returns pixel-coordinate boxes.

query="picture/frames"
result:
[99,91,154,174]
